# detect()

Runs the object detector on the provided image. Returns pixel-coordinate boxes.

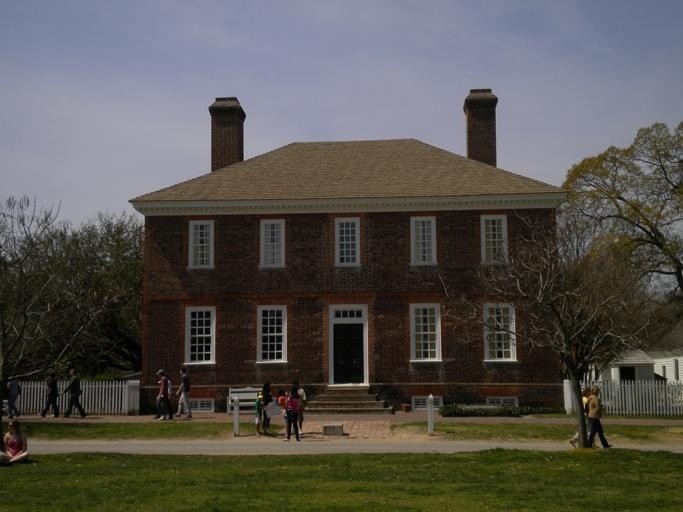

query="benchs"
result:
[226,386,272,416]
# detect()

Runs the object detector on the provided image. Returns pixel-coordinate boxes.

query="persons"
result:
[586,386,614,449]
[254,381,306,441]
[156,369,168,421]
[6,379,21,419]
[153,373,174,419]
[0,419,29,466]
[60,368,88,418]
[175,367,192,418]
[568,390,600,449]
[37,373,60,417]
